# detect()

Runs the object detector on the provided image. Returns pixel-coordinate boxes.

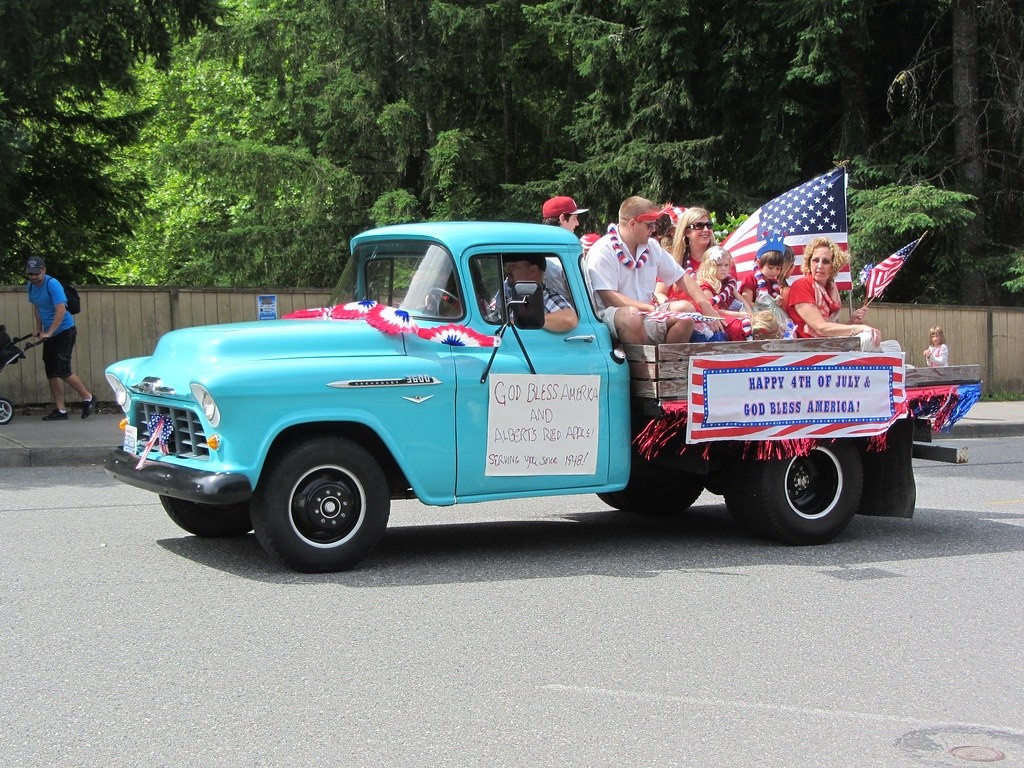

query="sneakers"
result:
[43,408,68,420]
[81,394,98,418]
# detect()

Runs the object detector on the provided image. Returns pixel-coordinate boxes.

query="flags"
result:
[717,167,855,291]
[638,311,716,322]
[863,238,919,299]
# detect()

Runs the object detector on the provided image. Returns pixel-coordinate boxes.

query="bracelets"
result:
[850,323,855,337]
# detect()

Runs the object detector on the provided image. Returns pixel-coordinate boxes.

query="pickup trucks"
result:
[106,220,982,574]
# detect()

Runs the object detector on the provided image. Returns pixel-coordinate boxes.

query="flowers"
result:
[710,249,724,260]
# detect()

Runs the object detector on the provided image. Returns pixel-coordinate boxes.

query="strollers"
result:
[0,331,46,425]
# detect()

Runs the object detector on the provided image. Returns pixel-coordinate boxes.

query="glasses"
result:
[28,273,41,276]
[686,222,713,230]
[642,221,655,230]
[810,257,833,266]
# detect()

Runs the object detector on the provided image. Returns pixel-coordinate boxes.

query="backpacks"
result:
[30,278,80,315]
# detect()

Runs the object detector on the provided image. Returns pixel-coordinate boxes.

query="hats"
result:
[25,257,44,274]
[504,252,547,272]
[542,197,590,218]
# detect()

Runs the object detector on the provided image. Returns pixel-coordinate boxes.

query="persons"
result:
[539,196,601,319]
[585,195,729,345]
[25,256,99,420]
[581,204,883,351]
[481,250,577,333]
[394,255,491,319]
[923,327,949,367]
